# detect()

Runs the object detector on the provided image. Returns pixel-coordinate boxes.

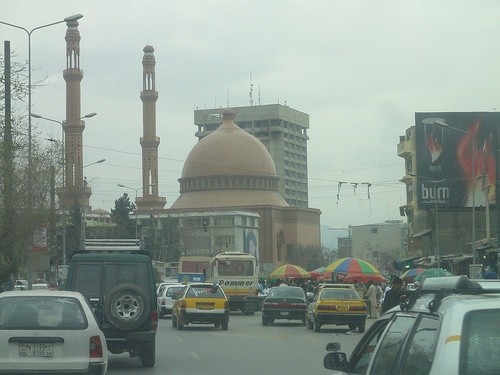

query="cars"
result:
[261,286,307,325]
[306,287,368,334]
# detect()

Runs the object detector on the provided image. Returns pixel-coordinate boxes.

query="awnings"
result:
[413,230,431,237]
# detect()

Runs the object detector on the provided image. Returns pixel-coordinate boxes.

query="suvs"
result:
[321,275,500,375]
[0,290,108,375]
[156,282,186,318]
[65,252,158,366]
[1,279,48,290]
[170,283,230,330]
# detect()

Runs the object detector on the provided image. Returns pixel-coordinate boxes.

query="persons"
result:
[383,278,402,313]
[484,268,496,279]
[258,279,383,319]
[245,232,258,266]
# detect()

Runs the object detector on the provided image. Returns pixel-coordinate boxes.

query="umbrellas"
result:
[325,257,380,275]
[309,267,332,277]
[343,273,385,282]
[400,268,455,284]
[270,264,311,278]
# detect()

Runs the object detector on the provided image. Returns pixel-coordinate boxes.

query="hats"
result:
[393,277,402,283]
[485,266,494,272]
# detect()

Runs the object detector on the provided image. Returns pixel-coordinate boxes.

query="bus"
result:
[205,252,260,314]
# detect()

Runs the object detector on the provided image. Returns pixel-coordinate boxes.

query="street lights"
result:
[1,14,84,116]
[117,182,156,238]
[435,120,476,265]
[29,112,98,264]
[81,159,106,167]
